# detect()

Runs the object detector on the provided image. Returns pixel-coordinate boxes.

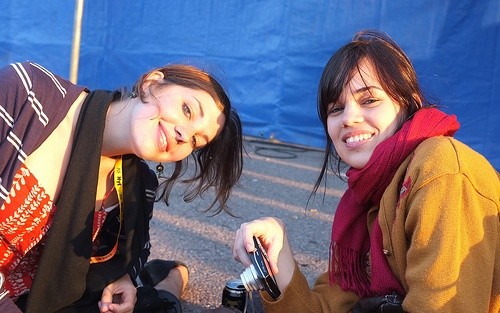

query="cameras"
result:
[238,236,281,300]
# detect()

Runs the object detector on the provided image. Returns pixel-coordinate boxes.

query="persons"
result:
[234,29,500,313]
[0,61,242,313]
